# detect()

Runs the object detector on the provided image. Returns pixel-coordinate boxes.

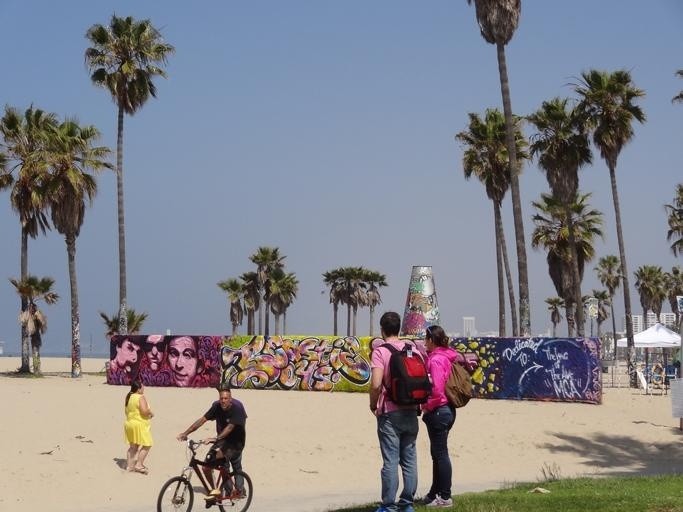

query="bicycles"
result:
[156,435,254,512]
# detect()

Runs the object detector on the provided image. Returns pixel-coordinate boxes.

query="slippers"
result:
[204,492,223,508]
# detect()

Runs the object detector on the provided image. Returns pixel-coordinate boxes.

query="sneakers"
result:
[422,492,453,508]
[378,504,415,512]
[224,487,247,499]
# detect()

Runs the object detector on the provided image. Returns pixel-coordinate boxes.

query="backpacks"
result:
[379,343,431,404]
[433,351,472,408]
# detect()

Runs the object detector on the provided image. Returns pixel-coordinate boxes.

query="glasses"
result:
[142,342,166,353]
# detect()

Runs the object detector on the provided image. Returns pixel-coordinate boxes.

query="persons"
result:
[420,326,461,509]
[123,381,154,474]
[141,335,168,372]
[367,311,432,512]
[165,335,205,387]
[211,382,248,499]
[109,336,145,384]
[176,390,246,499]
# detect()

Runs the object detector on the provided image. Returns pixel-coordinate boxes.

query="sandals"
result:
[127,465,149,473]
